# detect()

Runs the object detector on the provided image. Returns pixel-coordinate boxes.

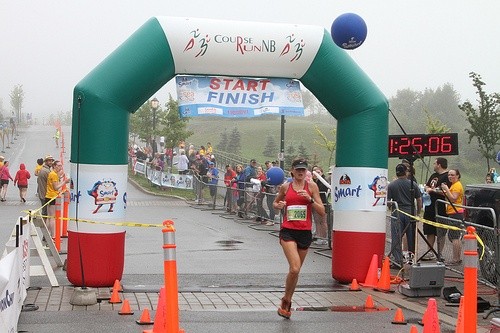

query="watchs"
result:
[310,199,314,204]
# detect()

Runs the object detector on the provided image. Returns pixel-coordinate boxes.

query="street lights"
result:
[151,97,159,158]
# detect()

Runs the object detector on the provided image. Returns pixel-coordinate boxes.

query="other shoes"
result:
[445,260,463,266]
[22,198,26,202]
[226,206,274,226]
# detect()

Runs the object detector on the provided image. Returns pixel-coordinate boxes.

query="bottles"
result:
[423,192,431,206]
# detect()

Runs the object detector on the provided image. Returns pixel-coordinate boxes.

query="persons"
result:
[224,159,332,244]
[423,158,453,261]
[273,158,326,318]
[128,142,219,203]
[34,156,70,223]
[14,163,30,202]
[441,169,464,266]
[386,159,422,269]
[0,156,13,202]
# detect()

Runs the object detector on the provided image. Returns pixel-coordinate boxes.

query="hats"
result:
[43,156,55,162]
[292,162,307,170]
[209,162,214,165]
[251,159,257,163]
[396,164,407,174]
[265,160,271,164]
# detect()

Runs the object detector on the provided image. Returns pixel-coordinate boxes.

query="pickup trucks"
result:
[462,182,500,286]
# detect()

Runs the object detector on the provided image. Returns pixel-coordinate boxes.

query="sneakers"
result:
[277,297,289,316]
[437,256,445,262]
[284,301,292,318]
[311,230,328,245]
[420,252,434,259]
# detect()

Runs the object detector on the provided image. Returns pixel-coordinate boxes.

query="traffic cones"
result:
[359,255,380,288]
[118,299,135,315]
[349,278,363,291]
[391,307,407,325]
[59,152,70,194]
[135,287,185,333]
[408,297,441,333]
[363,295,376,309]
[108,289,122,304]
[372,256,395,293]
[109,279,124,293]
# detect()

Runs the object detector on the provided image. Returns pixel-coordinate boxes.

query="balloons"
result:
[332,13,367,49]
